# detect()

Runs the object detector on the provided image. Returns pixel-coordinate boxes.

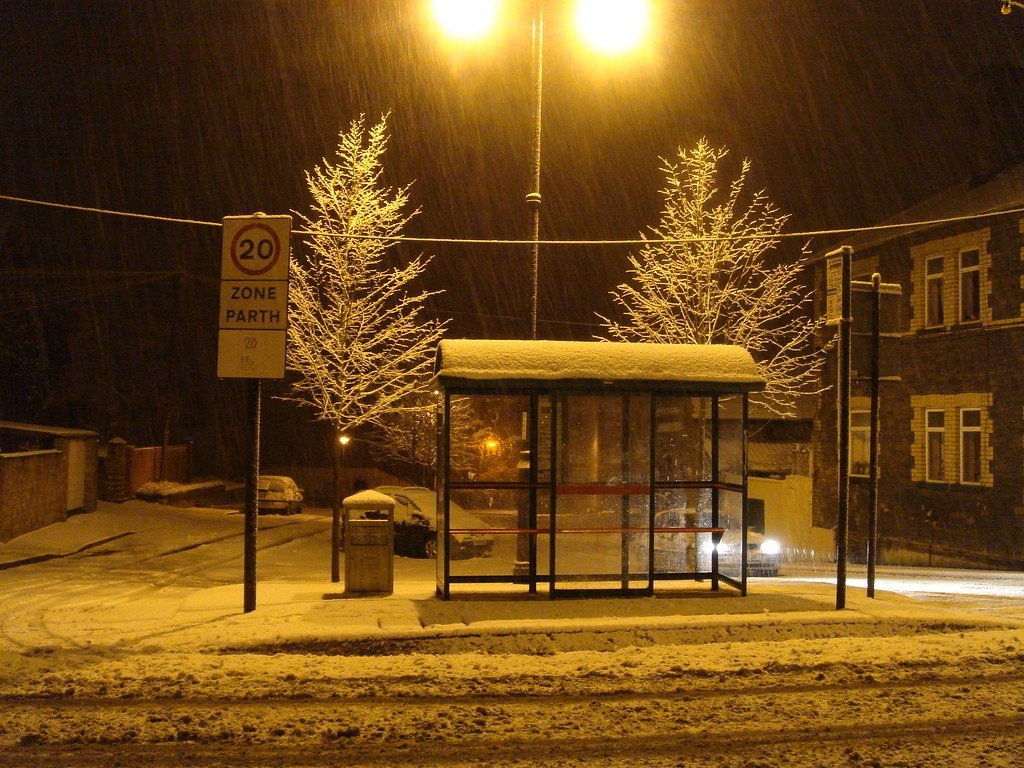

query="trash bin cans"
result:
[343,489,396,595]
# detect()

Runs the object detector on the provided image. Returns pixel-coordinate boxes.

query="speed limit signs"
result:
[232,220,283,276]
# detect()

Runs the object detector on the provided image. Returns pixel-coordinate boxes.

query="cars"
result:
[339,484,495,559]
[635,508,784,579]
[258,475,304,516]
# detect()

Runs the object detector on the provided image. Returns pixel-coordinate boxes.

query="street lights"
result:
[339,433,350,497]
